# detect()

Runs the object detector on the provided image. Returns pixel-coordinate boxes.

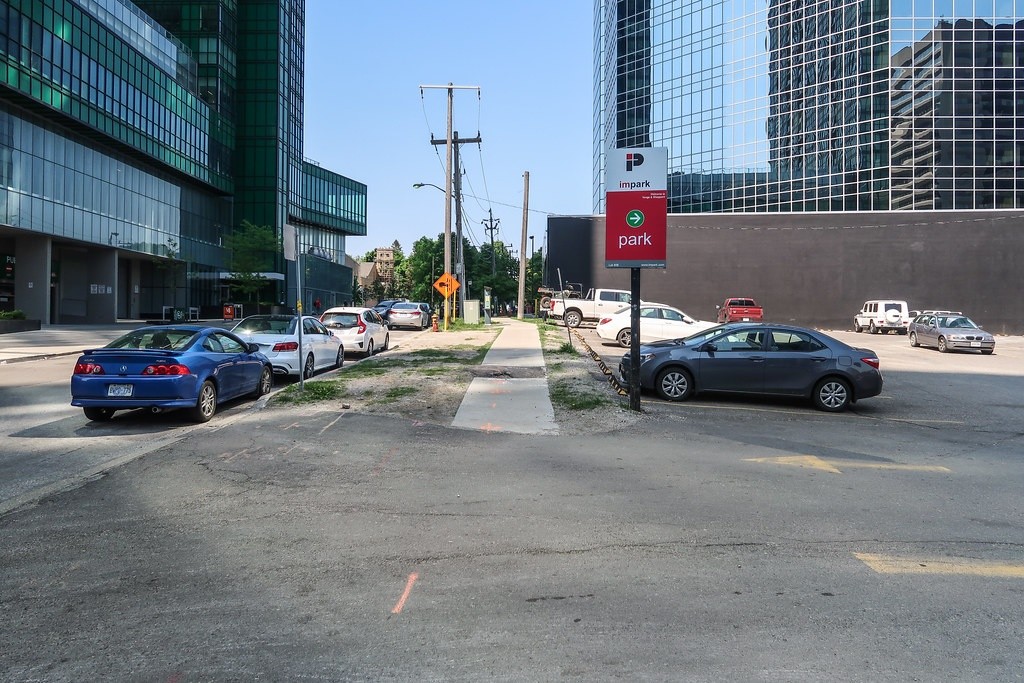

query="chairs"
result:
[760,332,776,351]
[260,322,272,334]
[152,336,172,350]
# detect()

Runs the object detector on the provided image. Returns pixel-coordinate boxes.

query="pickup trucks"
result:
[714,297,764,323]
[550,288,644,328]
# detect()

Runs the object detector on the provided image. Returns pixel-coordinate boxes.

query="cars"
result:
[373,300,403,329]
[909,313,996,355]
[317,306,390,357]
[596,301,721,348]
[421,302,435,317]
[230,314,344,380]
[389,303,429,331]
[617,322,884,412]
[68,324,274,423]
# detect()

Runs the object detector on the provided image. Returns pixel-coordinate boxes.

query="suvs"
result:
[853,299,909,335]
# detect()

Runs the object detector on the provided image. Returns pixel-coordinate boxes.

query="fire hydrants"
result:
[431,314,439,332]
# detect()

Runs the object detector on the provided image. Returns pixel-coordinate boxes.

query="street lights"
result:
[414,183,466,319]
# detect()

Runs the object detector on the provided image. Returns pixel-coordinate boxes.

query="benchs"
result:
[162,306,199,321]
